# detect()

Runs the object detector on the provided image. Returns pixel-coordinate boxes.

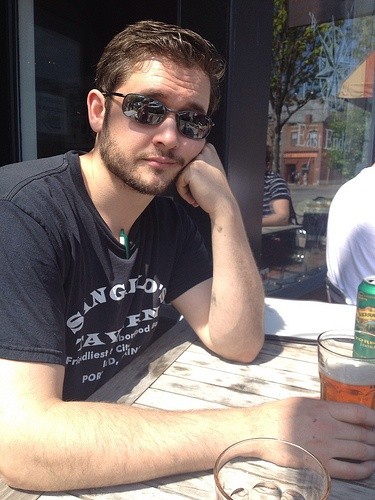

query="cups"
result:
[213,437,331,500]
[317,329,375,410]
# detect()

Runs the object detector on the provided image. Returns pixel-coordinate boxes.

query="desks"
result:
[0,298,375,500]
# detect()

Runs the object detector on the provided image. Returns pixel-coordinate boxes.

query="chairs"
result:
[262,195,332,303]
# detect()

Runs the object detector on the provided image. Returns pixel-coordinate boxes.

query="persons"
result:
[259,144,297,264]
[0,19,375,494]
[325,161,375,307]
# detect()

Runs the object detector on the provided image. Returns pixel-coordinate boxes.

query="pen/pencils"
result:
[117,225,132,262]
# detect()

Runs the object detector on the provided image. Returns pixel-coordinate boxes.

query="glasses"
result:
[99,93,215,140]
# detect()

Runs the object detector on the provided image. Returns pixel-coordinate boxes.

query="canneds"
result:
[352,276,375,365]
